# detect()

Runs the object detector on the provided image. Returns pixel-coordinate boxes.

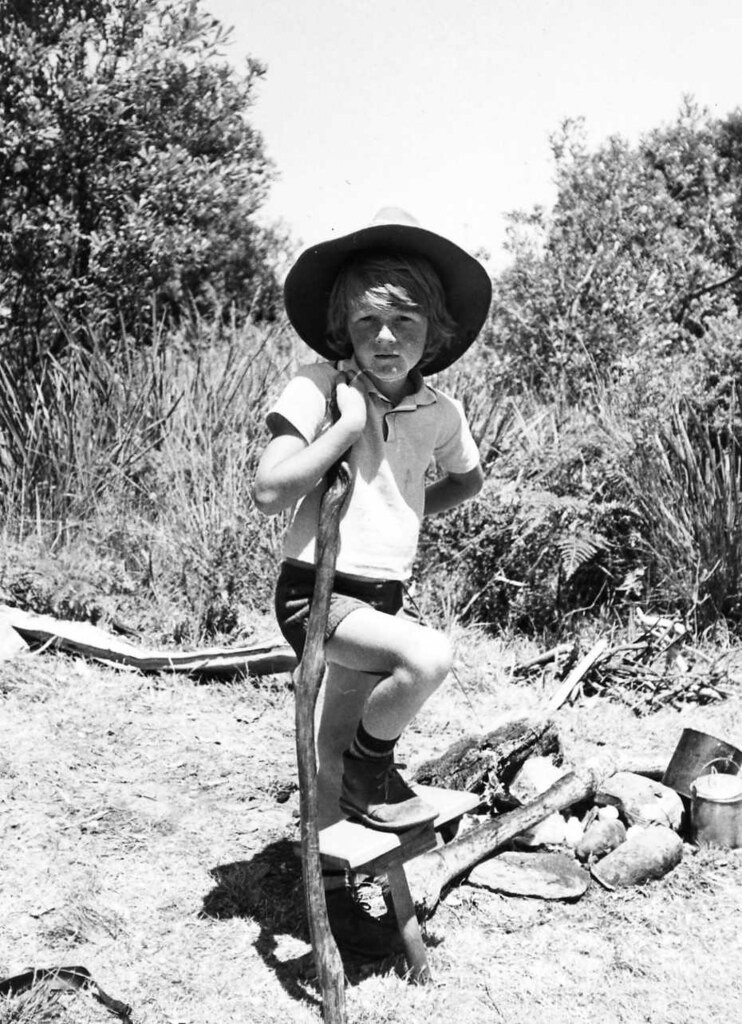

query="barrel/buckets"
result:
[689,757,742,848]
[662,727,742,801]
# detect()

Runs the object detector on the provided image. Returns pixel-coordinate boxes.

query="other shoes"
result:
[323,886,405,957]
[337,748,440,830]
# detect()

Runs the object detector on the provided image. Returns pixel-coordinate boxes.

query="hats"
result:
[283,205,493,375]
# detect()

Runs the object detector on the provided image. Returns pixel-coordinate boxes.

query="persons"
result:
[251,209,492,960]
